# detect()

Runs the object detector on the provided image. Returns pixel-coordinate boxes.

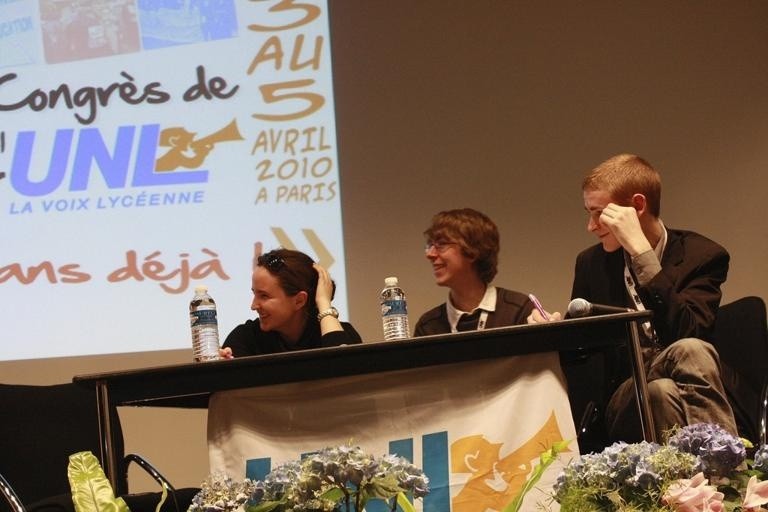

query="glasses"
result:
[424,239,458,252]
[263,253,285,273]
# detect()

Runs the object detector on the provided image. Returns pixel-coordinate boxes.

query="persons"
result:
[414,208,540,336]
[527,154,739,445]
[217,248,362,359]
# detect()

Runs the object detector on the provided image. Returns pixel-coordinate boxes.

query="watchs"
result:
[316,307,340,322]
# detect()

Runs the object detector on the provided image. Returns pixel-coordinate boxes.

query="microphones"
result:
[568,297,636,318]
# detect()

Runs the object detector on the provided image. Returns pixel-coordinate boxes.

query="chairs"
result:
[576,297,767,462]
[0,381,179,512]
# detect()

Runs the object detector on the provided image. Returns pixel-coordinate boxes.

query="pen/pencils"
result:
[528,293,549,322]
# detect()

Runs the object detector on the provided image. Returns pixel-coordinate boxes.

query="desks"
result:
[72,309,659,510]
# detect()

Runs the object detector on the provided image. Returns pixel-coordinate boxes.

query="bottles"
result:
[189,287,220,359]
[378,277,409,341]
